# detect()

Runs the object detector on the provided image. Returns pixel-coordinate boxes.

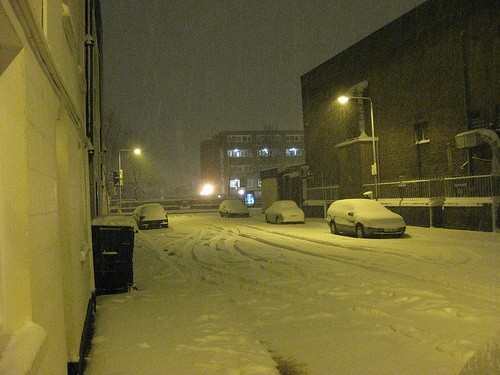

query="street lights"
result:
[339,93,381,206]
[118,148,141,213]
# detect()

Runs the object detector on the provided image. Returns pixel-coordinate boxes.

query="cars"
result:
[328,196,407,238]
[265,200,305,225]
[218,198,250,219]
[131,205,144,220]
[136,204,169,229]
